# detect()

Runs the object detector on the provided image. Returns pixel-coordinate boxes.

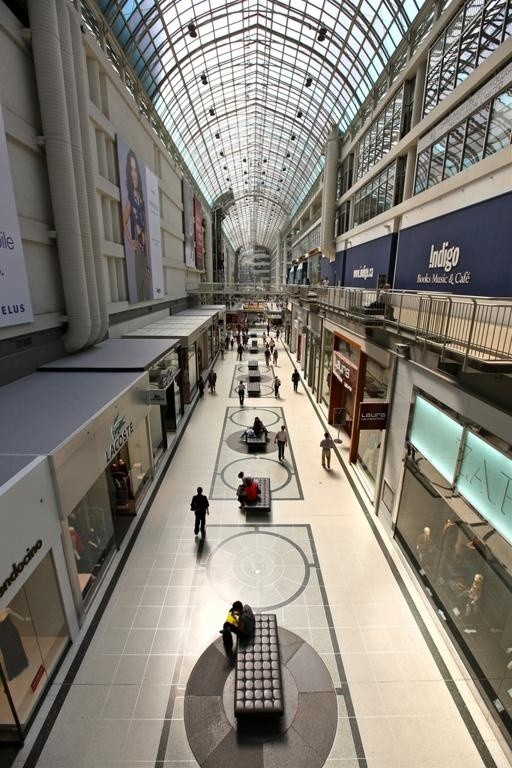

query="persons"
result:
[109,461,128,506]
[271,376,281,397]
[274,424,288,461]
[85,528,106,554]
[118,459,133,498]
[377,283,399,322]
[197,374,205,397]
[237,476,259,509]
[215,313,285,369]
[190,486,210,540]
[320,431,335,468]
[208,368,217,391]
[236,471,253,495]
[122,146,152,304]
[69,525,86,561]
[238,379,246,405]
[253,416,268,440]
[0,604,33,681]
[291,368,300,391]
[241,426,255,445]
[219,601,256,647]
[454,571,483,618]
[202,276,330,312]
[416,526,434,566]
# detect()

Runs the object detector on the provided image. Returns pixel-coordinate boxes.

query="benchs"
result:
[247,333,258,353]
[246,360,261,397]
[243,477,271,514]
[232,613,285,743]
[245,431,268,454]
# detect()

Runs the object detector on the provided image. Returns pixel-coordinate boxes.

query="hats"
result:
[230,601,242,612]
